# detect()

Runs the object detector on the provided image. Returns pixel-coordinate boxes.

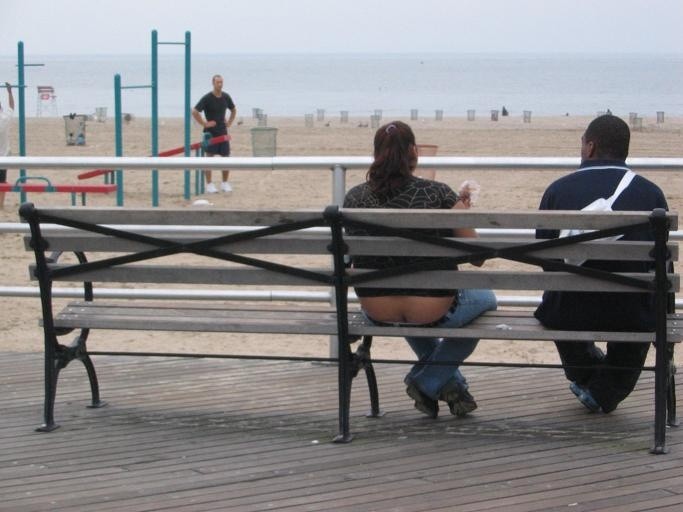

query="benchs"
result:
[17,203,683,454]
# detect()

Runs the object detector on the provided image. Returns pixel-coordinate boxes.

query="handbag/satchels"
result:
[559,199,624,264]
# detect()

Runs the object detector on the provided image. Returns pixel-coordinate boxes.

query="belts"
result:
[356,296,457,328]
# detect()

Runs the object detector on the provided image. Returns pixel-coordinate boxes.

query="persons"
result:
[191,74,237,194]
[346,119,496,421]
[0,82,15,209]
[530,113,673,414]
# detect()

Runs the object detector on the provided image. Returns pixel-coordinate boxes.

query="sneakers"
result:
[569,378,602,410]
[204,181,232,192]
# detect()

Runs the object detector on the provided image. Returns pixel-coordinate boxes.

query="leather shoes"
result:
[405,374,477,416]
